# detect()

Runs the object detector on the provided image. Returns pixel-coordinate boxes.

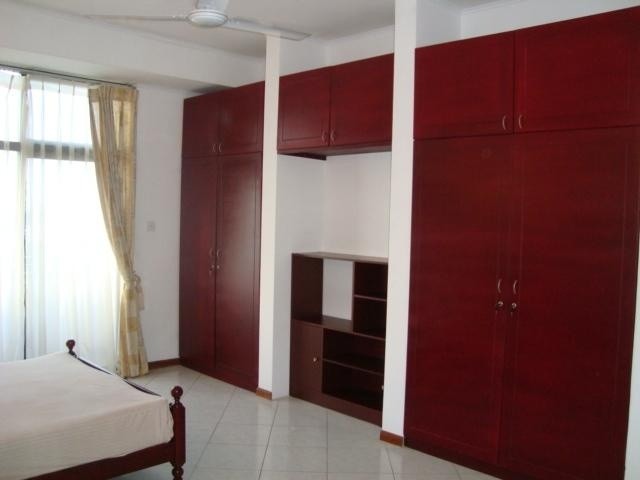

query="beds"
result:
[0,339,186,480]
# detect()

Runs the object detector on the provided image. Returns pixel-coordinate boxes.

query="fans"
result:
[83,0,312,42]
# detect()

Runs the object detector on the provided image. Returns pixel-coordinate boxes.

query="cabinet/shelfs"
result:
[289,251,388,429]
[277,53,394,156]
[180,80,264,393]
